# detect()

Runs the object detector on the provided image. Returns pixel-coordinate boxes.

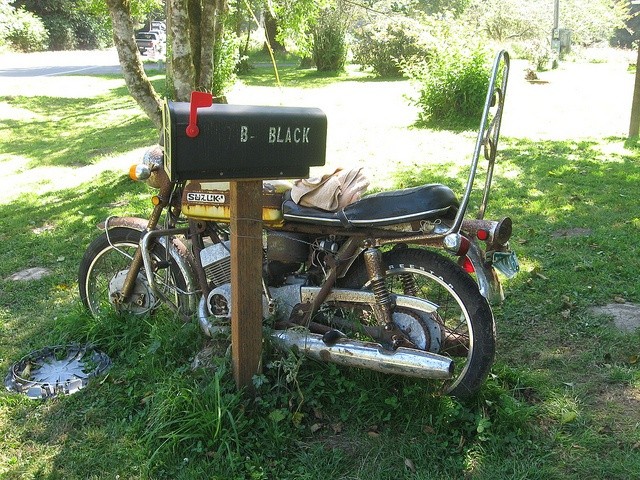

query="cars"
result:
[135,33,162,56]
[149,30,166,43]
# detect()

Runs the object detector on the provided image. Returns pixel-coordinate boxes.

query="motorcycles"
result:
[78,49,512,407]
